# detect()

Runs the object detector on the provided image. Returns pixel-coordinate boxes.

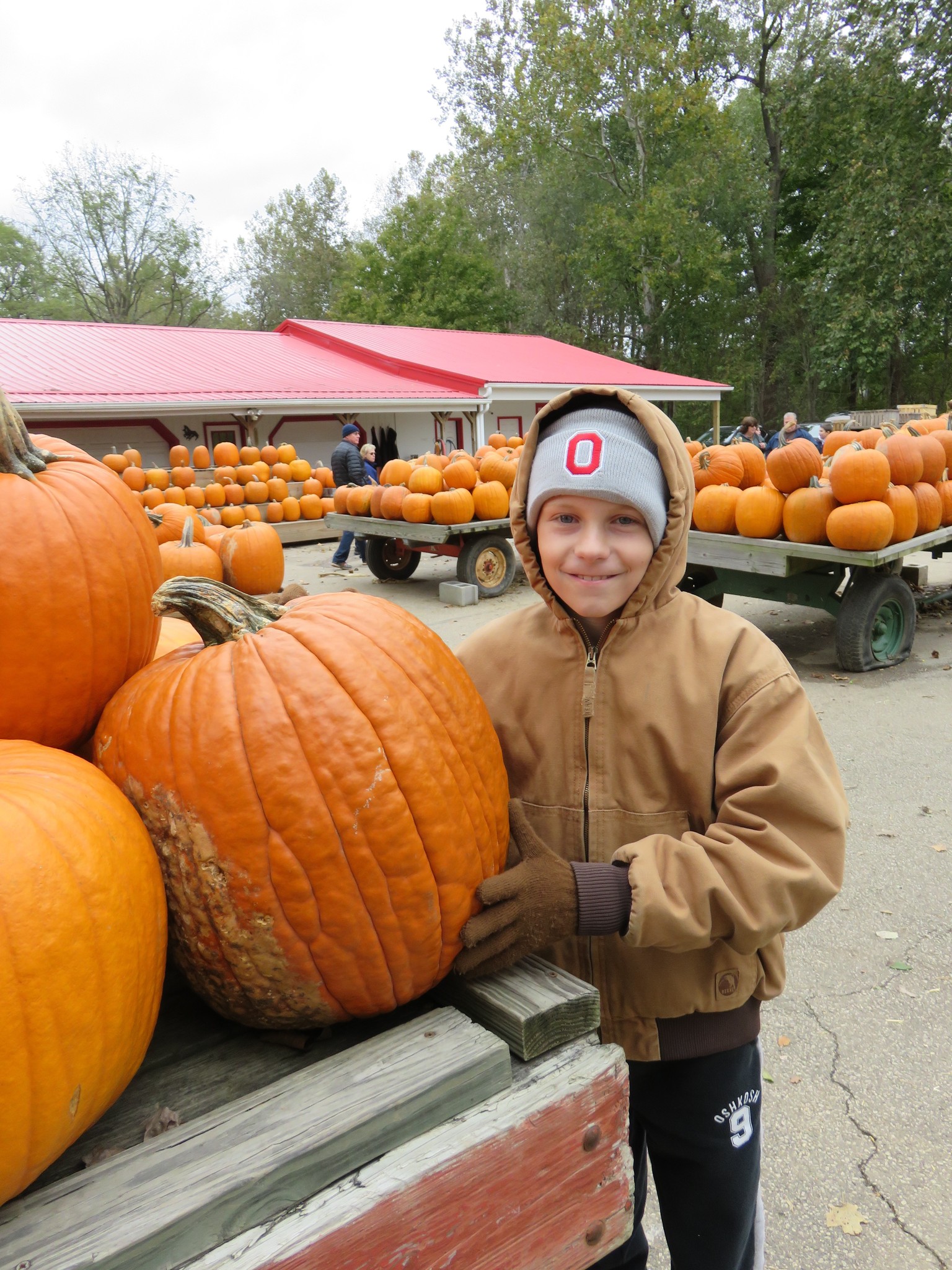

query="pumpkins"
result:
[683,413,952,550]
[100,437,336,528]
[0,387,165,750]
[0,737,168,1211]
[144,503,285,596]
[94,574,512,1026]
[72,616,202,763]
[332,430,530,524]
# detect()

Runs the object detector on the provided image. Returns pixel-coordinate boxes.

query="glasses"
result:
[750,424,757,428]
[370,452,376,455]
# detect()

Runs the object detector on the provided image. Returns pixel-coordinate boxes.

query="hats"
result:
[342,423,360,438]
[524,405,667,551]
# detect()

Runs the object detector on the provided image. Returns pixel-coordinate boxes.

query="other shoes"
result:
[331,560,353,569]
[353,548,363,558]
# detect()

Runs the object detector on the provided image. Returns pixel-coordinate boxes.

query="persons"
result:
[451,384,846,1270]
[330,424,379,570]
[732,413,833,463]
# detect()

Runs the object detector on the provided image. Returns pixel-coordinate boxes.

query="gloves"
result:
[445,798,578,982]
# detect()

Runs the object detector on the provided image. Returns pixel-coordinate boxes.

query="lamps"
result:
[248,408,263,421]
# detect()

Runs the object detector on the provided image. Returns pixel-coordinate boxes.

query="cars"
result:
[696,425,772,454]
[798,410,856,445]
